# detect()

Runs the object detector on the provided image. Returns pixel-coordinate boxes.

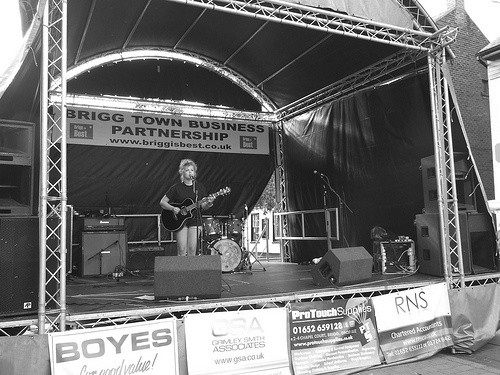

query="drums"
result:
[203,218,220,239]
[205,238,243,272]
[225,219,241,238]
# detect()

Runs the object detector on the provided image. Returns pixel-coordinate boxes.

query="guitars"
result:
[161,187,231,232]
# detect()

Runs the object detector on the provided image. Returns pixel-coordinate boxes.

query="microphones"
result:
[314,170,323,176]
[190,173,195,180]
[218,252,222,255]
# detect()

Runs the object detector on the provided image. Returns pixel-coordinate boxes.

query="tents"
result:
[0,0,500,335]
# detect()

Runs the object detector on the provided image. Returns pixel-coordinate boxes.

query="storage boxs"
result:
[373,239,415,275]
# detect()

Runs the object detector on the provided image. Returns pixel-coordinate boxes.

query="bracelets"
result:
[207,203,213,207]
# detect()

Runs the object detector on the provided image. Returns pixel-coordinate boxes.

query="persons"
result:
[160,159,213,256]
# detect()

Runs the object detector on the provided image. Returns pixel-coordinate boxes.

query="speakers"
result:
[0,119,60,316]
[79,231,129,278]
[153,254,222,300]
[417,153,495,277]
[309,246,373,287]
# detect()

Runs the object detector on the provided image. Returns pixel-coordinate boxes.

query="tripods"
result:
[240,204,266,272]
